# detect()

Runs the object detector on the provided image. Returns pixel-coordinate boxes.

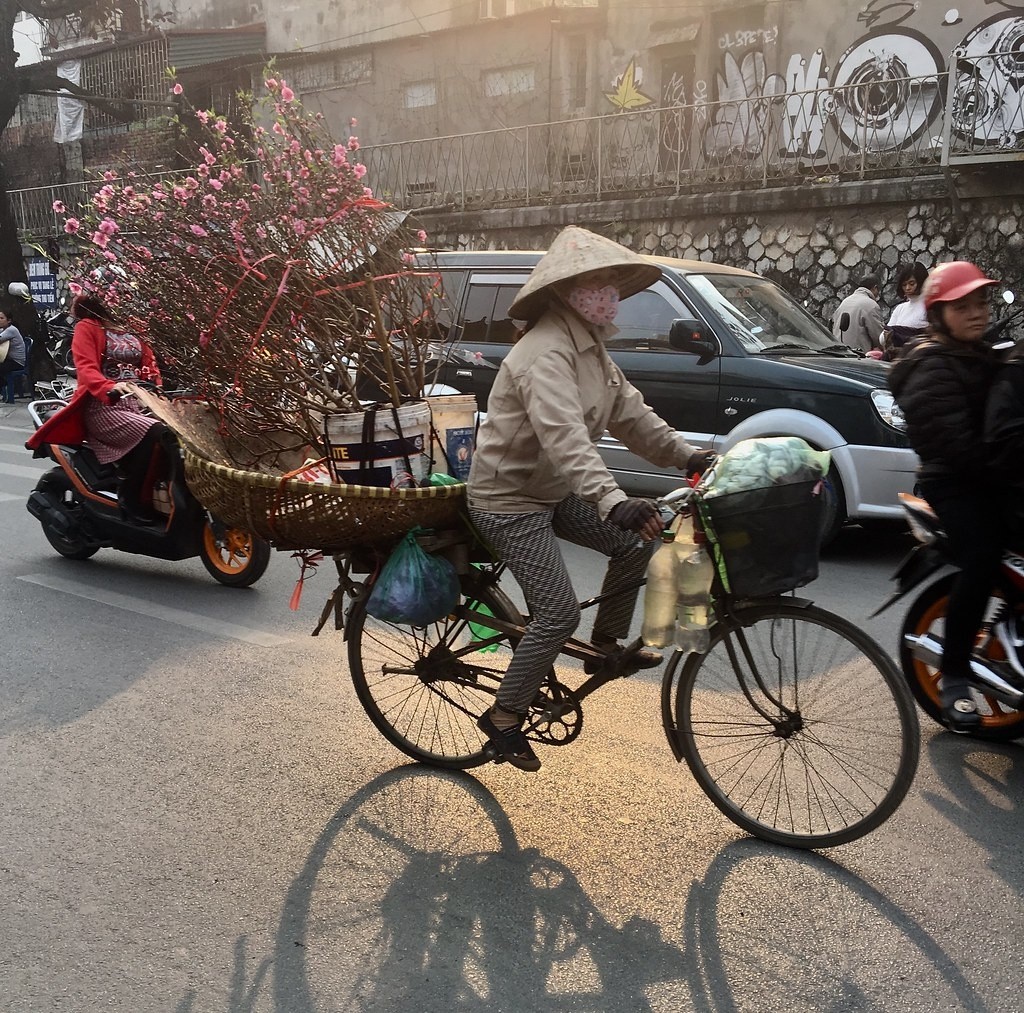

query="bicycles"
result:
[303,427,920,854]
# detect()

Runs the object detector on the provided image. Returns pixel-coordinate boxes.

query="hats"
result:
[507,224,663,320]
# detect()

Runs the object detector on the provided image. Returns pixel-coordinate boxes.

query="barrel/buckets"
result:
[420,394,478,484]
[320,399,430,490]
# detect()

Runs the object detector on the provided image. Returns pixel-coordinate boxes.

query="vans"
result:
[353,250,927,548]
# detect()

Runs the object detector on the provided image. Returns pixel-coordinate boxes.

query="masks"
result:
[567,285,620,326]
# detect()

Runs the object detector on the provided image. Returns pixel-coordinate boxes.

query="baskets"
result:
[698,477,825,597]
[181,445,469,551]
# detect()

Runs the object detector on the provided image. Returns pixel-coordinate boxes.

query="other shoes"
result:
[939,677,983,734]
[117,495,158,525]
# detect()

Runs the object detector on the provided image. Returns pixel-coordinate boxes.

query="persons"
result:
[833,273,882,355]
[70,266,169,526]
[466,247,720,772]
[0,310,26,388]
[879,261,1024,734]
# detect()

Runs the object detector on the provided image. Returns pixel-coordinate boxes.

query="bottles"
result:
[669,512,700,651]
[641,530,680,649]
[678,531,715,655]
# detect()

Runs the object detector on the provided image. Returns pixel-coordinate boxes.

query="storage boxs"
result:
[317,397,432,486]
[411,393,478,482]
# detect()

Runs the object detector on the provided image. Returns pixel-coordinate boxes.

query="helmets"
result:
[922,261,1001,310]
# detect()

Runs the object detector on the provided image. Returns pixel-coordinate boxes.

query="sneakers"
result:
[993,616,1024,677]
[584,642,664,675]
[476,708,542,772]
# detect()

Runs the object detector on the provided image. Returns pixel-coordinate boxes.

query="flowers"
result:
[25,59,453,467]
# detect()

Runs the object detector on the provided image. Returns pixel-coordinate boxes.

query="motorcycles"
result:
[872,480,1024,744]
[23,353,274,587]
